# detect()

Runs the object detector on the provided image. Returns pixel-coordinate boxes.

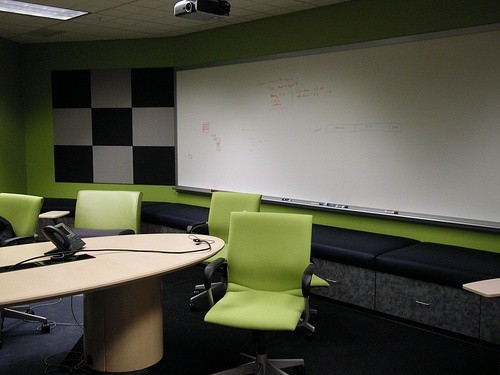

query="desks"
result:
[0,233,225,375]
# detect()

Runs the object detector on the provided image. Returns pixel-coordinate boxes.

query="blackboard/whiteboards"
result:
[174,22,499,231]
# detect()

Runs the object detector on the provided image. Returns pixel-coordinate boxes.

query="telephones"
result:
[42,221,85,254]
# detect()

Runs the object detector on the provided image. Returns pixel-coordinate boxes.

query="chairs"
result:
[75,190,143,233]
[298,270,341,334]
[189,191,263,303]
[202,211,333,375]
[0,192,50,332]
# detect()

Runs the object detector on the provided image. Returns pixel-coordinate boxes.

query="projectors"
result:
[173,0,231,19]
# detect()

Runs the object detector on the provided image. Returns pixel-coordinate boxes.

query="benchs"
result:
[39,195,500,344]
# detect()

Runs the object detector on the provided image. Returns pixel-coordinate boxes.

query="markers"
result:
[318,203,349,208]
[282,198,290,201]
[385,210,398,214]
